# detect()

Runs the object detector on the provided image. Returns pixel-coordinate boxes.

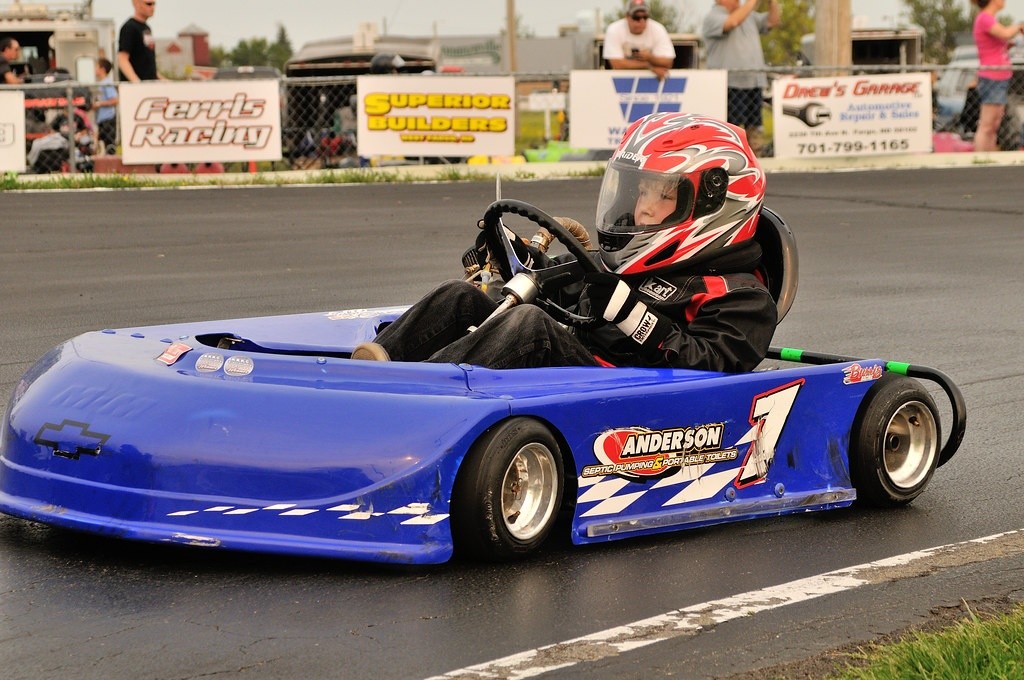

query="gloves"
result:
[475,219,536,276]
[579,273,672,360]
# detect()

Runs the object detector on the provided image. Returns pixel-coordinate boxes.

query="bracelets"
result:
[95,101,100,107]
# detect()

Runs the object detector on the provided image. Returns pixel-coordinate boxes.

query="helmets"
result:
[595,111,766,275]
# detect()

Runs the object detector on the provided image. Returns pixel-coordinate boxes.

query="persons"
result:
[0,36,29,84]
[970,0,1024,154]
[89,57,119,155]
[117,0,168,83]
[951,66,981,139]
[701,0,782,159]
[921,68,938,132]
[601,0,676,79]
[344,110,778,375]
[25,101,88,171]
[369,51,405,74]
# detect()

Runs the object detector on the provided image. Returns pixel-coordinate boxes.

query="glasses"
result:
[145,2,155,6]
[632,14,648,20]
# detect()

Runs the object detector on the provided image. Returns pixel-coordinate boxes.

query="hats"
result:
[625,0,652,15]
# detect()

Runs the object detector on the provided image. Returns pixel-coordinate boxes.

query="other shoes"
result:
[350,343,393,361]
